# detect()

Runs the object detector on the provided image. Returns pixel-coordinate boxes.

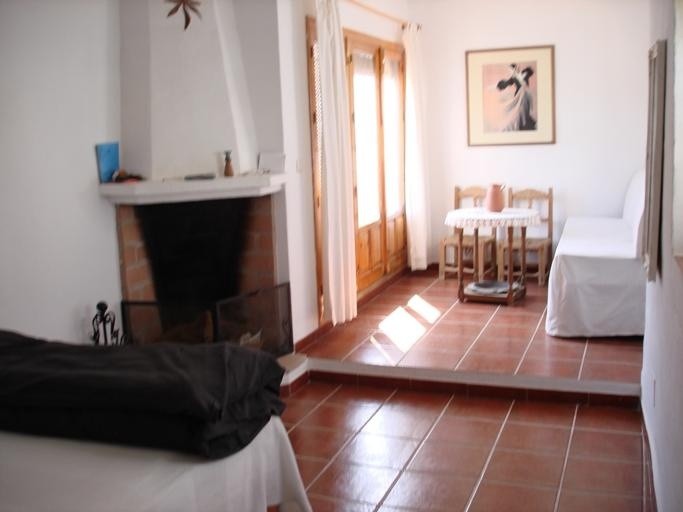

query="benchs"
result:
[546,171,649,339]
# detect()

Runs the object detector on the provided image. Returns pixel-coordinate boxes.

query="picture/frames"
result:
[464,43,555,147]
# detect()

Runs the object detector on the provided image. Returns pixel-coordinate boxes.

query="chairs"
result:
[437,184,555,285]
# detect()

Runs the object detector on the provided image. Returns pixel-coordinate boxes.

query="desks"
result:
[446,207,539,308]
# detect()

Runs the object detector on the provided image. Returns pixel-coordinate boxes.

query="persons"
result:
[489,61,534,131]
